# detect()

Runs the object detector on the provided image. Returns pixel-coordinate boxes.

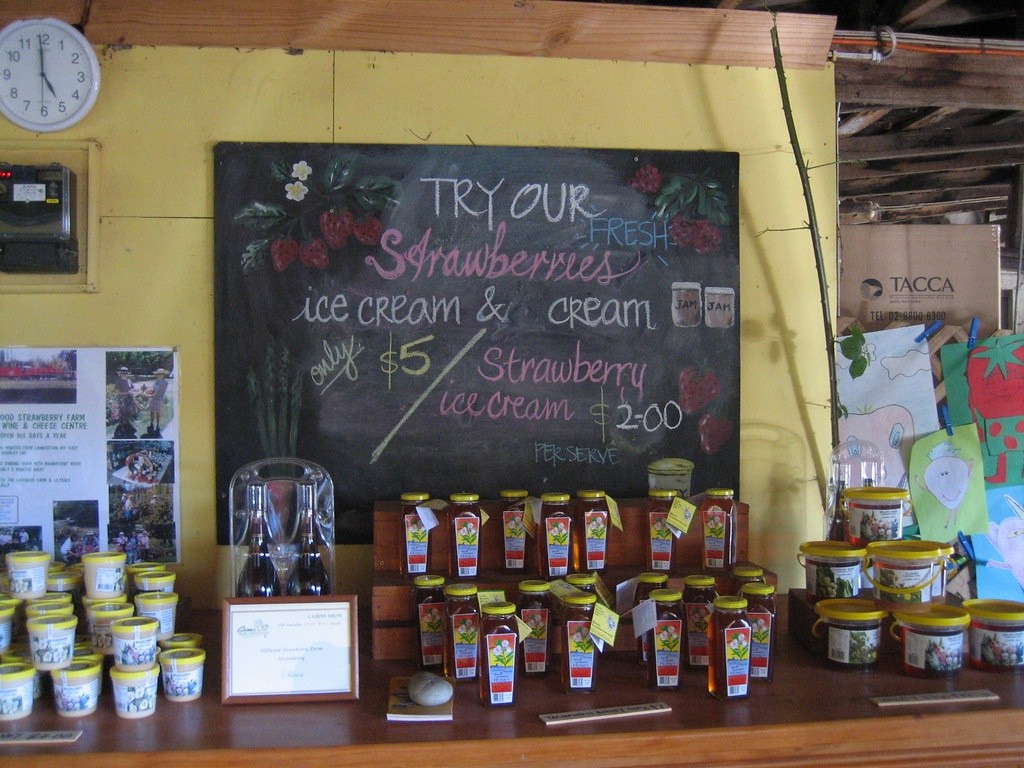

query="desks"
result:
[0,647,1024,768]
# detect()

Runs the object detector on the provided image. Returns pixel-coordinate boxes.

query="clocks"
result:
[0,16,101,134]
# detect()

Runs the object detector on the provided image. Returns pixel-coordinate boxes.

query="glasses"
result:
[121,373,127,375]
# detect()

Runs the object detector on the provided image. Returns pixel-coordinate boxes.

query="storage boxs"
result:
[607,499,646,566]
[475,582,522,608]
[374,500,401,572]
[672,509,703,566]
[735,501,751,564]
[596,620,638,653]
[713,565,778,597]
[372,626,418,660]
[786,585,903,658]
[480,500,506,569]
[429,510,450,571]
[372,585,419,622]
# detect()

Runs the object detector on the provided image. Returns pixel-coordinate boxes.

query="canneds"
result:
[396,488,778,708]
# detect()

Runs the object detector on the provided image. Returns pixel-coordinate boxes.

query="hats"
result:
[116,367,132,375]
[152,369,169,375]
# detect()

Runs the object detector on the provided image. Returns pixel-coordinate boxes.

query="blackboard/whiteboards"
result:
[213,140,743,547]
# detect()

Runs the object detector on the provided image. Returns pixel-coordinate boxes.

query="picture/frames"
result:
[221,594,361,706]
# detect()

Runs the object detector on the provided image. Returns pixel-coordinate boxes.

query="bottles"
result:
[538,492,572,580]
[397,491,434,580]
[450,492,482,577]
[577,490,610,573]
[700,488,739,571]
[286,509,330,597]
[499,489,528,573]
[411,566,777,708]
[647,489,677,575]
[828,481,849,540]
[864,479,873,486]
[237,514,280,598]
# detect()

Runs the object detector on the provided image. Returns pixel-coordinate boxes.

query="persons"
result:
[0,527,29,556]
[61,530,99,561]
[116,367,136,438]
[144,369,168,437]
[105,446,162,562]
